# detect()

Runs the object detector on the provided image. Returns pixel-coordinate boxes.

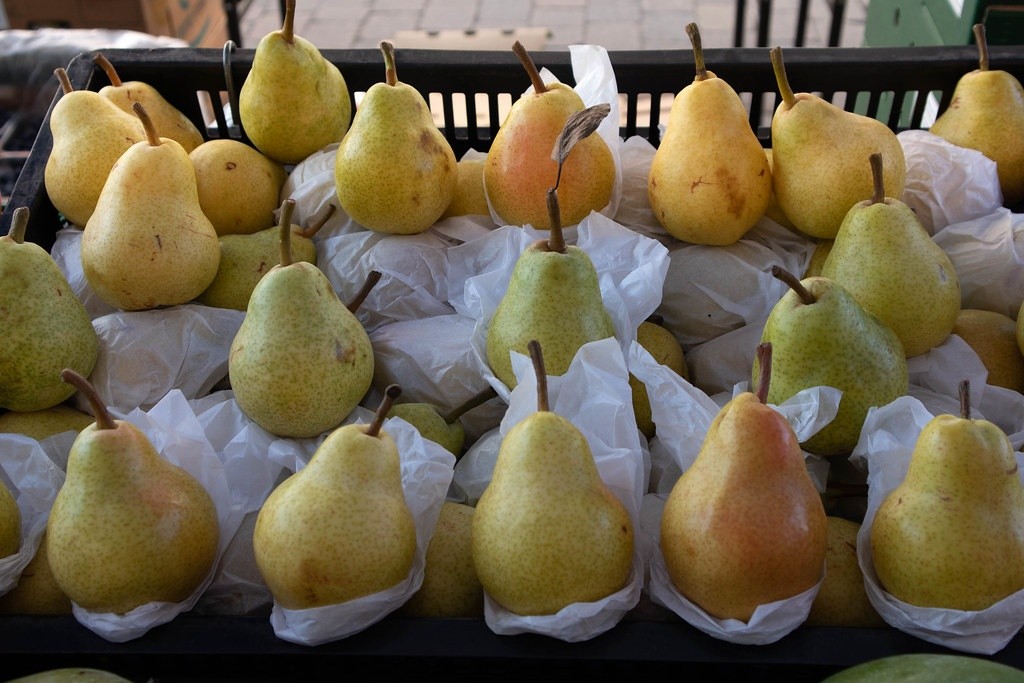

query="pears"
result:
[0,0,1024,683]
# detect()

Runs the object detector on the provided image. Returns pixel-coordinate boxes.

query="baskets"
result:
[0,42,1024,261]
